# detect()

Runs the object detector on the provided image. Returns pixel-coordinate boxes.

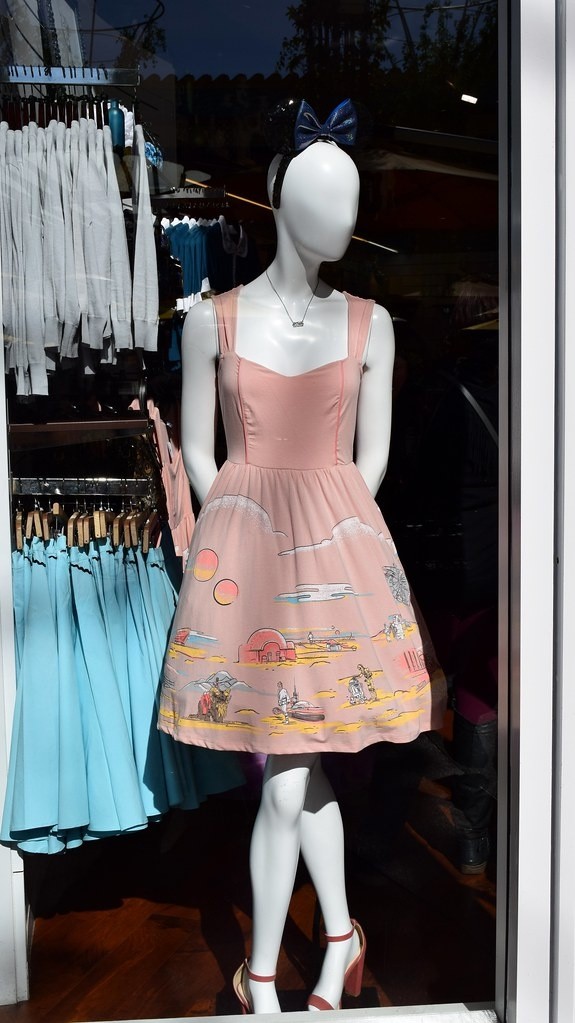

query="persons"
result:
[389,279,499,888]
[177,143,395,1013]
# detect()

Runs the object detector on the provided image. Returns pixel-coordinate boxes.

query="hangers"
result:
[0,65,148,130]
[12,466,161,554]
[157,185,242,239]
